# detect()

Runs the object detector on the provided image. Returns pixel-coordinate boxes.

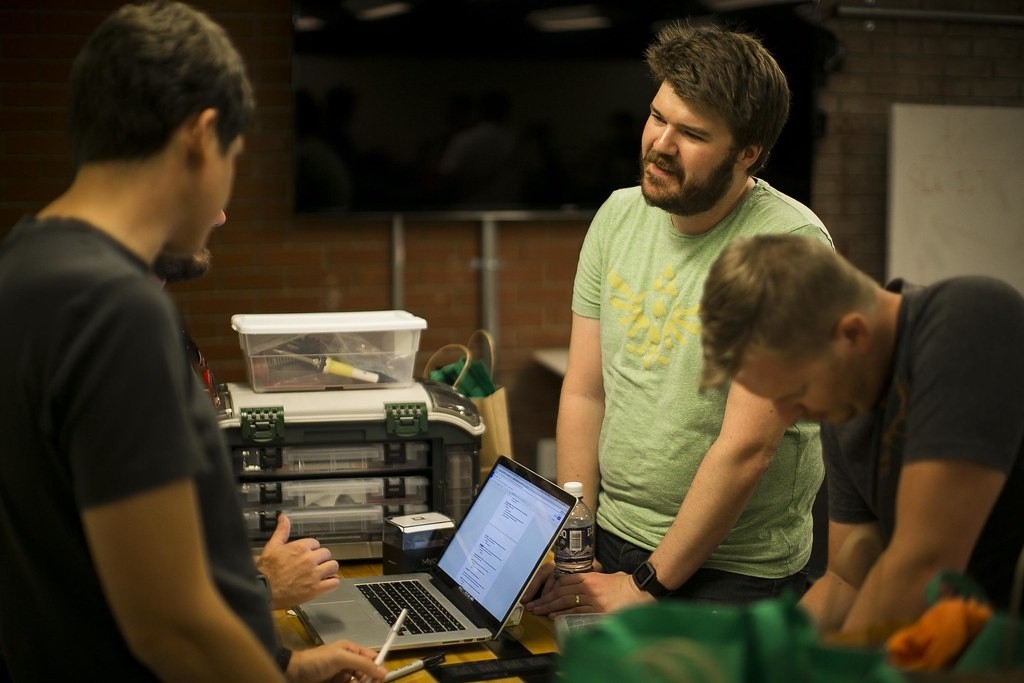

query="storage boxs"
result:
[231,309,427,393]
[381,512,456,576]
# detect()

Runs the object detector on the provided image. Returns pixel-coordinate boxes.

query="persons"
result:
[0,0,388,683]
[520,18,838,622]
[699,232,1023,647]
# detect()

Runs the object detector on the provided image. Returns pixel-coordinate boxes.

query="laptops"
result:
[296,455,580,655]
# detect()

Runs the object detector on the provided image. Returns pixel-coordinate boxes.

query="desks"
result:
[272,550,564,683]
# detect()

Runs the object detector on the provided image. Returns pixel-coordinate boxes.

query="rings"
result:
[574,594,581,607]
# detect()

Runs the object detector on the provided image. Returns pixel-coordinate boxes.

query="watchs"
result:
[632,560,674,603]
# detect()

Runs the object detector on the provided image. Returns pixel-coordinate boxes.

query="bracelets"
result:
[256,569,273,598]
[274,648,291,673]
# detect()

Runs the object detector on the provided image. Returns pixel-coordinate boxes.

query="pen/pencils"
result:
[384,651,447,682]
[364,608,407,682]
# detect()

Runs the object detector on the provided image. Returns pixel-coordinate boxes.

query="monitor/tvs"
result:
[288,0,712,221]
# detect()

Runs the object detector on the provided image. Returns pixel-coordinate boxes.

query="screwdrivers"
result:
[271,348,383,384]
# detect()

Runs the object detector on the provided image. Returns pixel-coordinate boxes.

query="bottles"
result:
[551,482,595,588]
